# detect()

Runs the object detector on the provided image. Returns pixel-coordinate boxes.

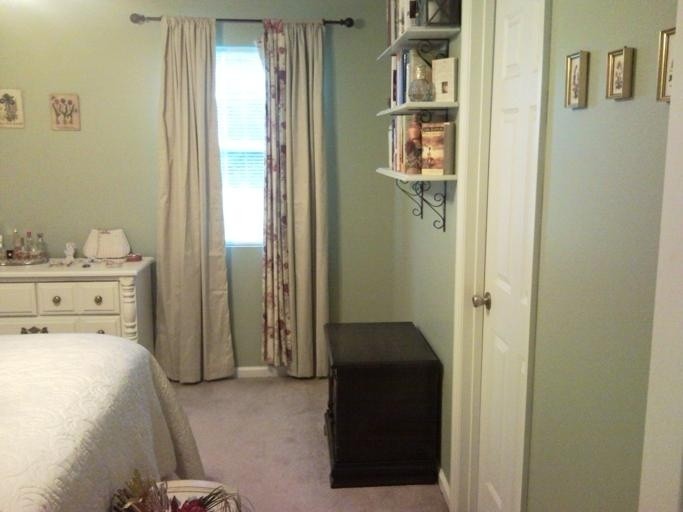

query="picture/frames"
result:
[565,50,588,109]
[656,27,676,102]
[606,47,634,100]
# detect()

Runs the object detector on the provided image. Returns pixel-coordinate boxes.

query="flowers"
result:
[109,460,251,512]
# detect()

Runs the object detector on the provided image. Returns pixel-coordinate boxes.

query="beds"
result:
[0,333,206,512]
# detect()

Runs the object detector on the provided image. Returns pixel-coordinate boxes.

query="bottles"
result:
[409,64,432,101]
[0,228,46,266]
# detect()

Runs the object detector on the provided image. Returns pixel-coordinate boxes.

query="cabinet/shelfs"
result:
[0,257,155,354]
[376,0,461,232]
[324,322,440,489]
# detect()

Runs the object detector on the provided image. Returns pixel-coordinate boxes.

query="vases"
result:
[148,479,236,512]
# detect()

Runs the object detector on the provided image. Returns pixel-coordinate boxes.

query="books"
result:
[385,2,456,176]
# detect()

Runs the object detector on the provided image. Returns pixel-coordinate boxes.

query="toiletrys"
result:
[36,230,46,250]
[12,227,21,250]
[3,229,11,249]
[0,234,3,247]
[22,232,32,251]
